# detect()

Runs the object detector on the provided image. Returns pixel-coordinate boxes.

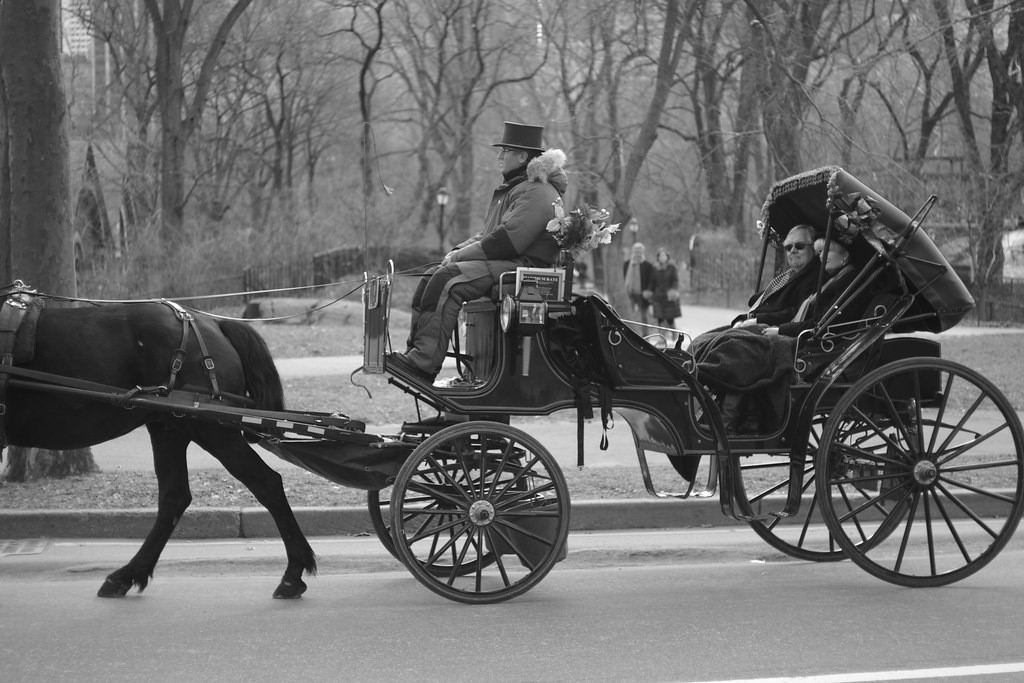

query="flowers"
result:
[546,199,622,253]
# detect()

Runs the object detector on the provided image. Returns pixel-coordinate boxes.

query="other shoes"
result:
[390,350,437,385]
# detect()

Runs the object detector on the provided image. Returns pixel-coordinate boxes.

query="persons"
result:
[623,243,652,335]
[686,224,869,437]
[384,121,567,384]
[643,250,681,340]
[576,257,586,289]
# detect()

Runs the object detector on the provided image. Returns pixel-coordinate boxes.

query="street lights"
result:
[434,187,450,257]
[629,217,639,243]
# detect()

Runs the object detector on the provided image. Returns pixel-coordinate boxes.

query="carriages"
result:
[0,164,1024,604]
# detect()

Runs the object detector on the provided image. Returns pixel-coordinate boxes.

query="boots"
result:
[700,392,742,435]
[738,400,760,434]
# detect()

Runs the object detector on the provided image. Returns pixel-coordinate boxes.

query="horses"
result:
[0,278,318,601]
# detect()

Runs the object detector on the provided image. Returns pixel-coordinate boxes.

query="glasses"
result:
[785,242,813,251]
[500,147,515,152]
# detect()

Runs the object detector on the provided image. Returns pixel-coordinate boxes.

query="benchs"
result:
[463,266,566,380]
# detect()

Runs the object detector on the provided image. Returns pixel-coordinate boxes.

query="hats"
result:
[491,121,545,152]
[817,210,860,259]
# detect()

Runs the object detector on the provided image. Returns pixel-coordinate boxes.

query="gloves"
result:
[441,249,458,266]
